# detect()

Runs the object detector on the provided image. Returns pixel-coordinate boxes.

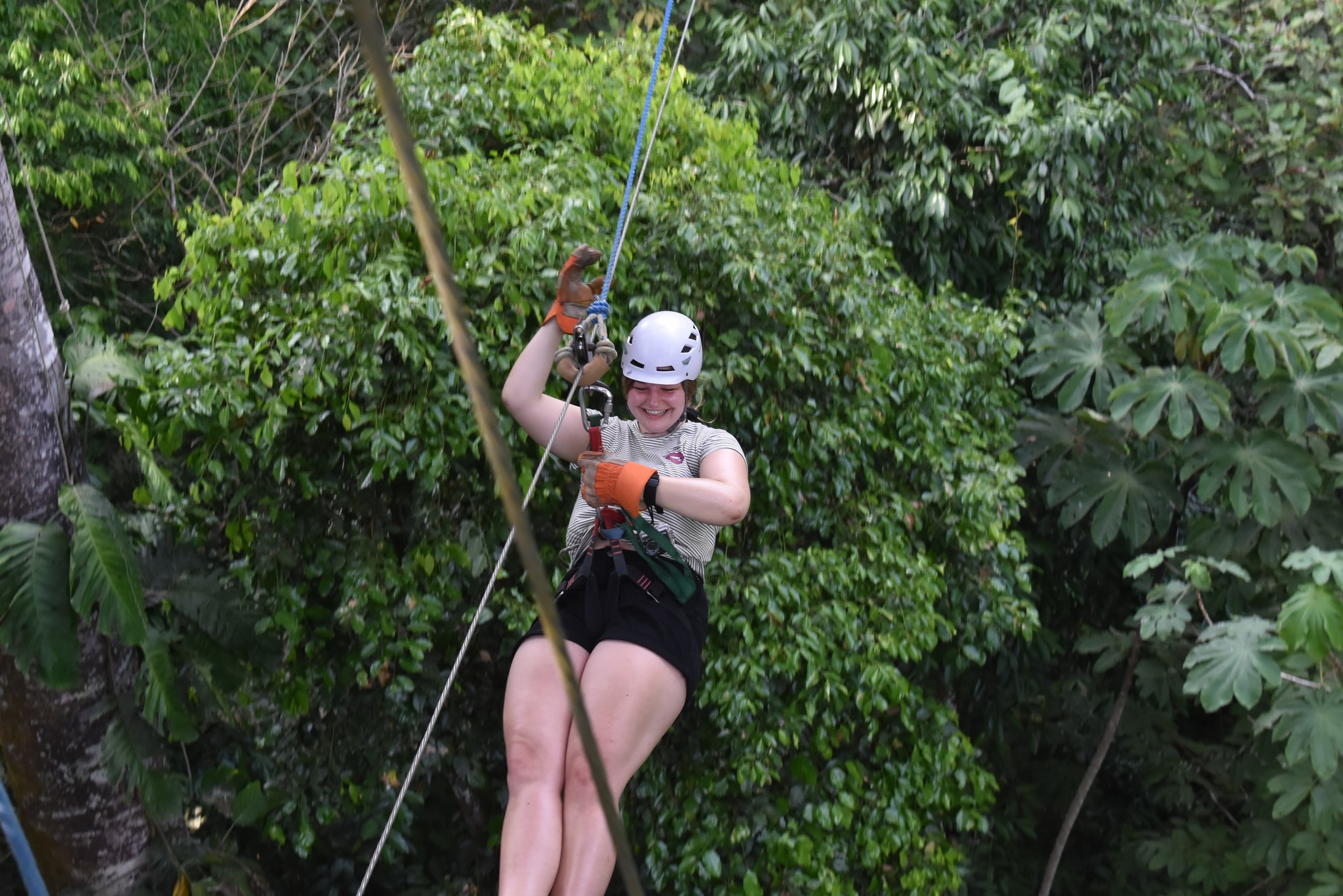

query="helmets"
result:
[621,310,703,385]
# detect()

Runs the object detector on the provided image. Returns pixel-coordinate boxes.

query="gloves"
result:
[541,243,615,335]
[578,451,658,517]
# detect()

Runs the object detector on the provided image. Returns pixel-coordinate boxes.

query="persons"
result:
[498,244,751,896]
